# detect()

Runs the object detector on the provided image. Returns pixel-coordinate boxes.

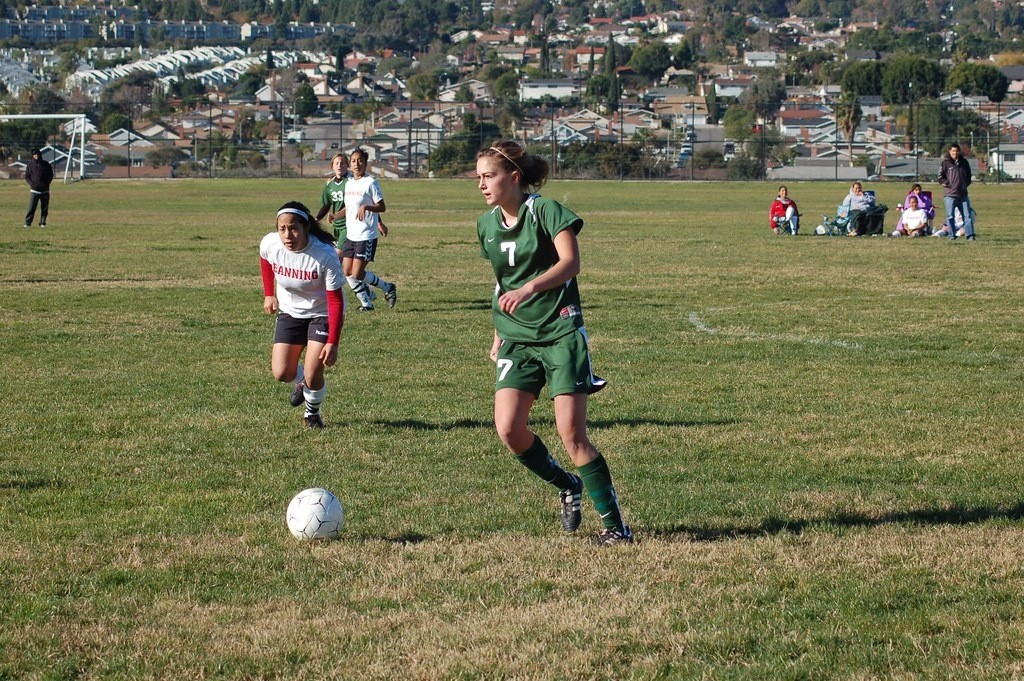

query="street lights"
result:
[293,97,304,133]
[667,126,681,161]
[239,117,252,144]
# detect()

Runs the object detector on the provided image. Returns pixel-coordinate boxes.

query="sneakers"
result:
[558,472,582,533]
[24,225,30,227]
[304,413,324,432]
[367,292,377,301]
[41,225,45,227]
[358,304,374,312]
[596,525,632,548]
[290,382,304,406]
[384,283,396,309]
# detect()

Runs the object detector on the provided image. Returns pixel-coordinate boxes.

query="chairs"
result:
[776,213,804,235]
[821,191,876,237]
[896,190,938,230]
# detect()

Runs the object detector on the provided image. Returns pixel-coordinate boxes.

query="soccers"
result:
[286,487,345,540]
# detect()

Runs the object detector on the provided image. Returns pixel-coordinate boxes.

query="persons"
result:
[258,200,346,430]
[769,186,800,236]
[24,148,53,227]
[887,183,935,237]
[475,140,631,546]
[931,144,976,242]
[841,182,870,237]
[315,146,397,313]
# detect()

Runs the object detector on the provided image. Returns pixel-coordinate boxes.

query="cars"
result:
[288,126,303,143]
[656,125,697,158]
